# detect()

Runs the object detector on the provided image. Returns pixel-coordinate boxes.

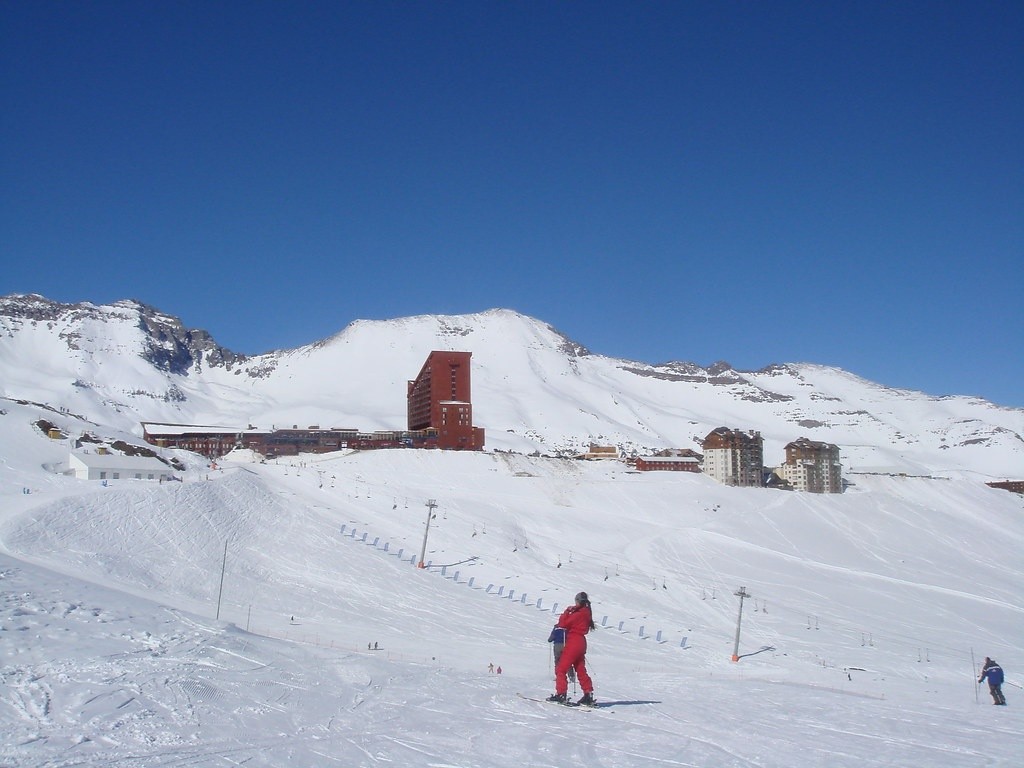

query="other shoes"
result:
[568,674,575,682]
[546,692,566,701]
[577,694,593,705]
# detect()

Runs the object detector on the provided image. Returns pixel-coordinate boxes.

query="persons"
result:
[551,592,595,703]
[548,624,574,682]
[978,657,1005,705]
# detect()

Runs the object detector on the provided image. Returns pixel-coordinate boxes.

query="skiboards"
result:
[516,692,616,714]
